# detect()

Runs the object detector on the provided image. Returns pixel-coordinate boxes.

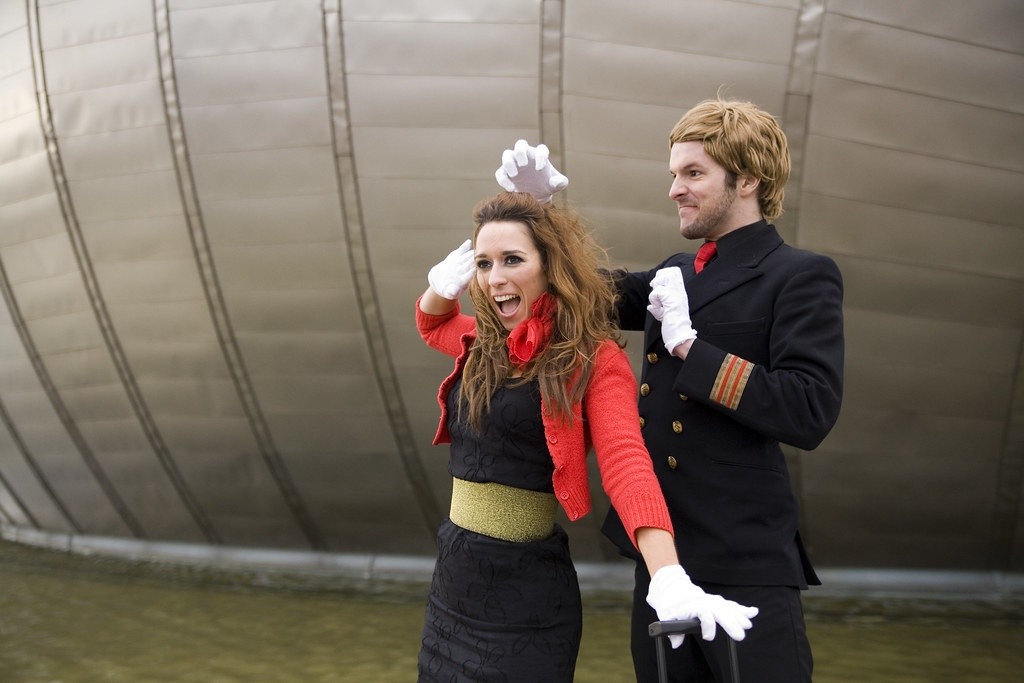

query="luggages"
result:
[648,619,742,683]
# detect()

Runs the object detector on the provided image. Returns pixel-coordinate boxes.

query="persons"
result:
[494,82,845,683]
[415,191,759,683]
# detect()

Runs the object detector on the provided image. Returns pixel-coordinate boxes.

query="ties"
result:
[694,241,716,272]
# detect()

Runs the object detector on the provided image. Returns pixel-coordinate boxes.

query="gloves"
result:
[645,265,699,358]
[645,563,759,650]
[426,237,474,300]
[495,136,571,209]
